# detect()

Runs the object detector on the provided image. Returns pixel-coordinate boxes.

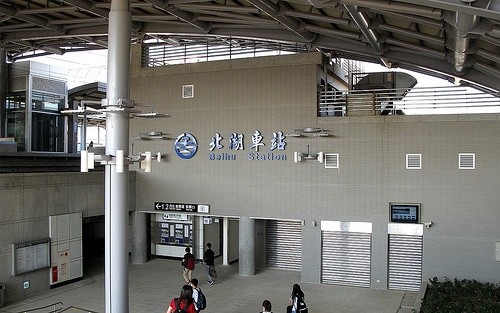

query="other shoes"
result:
[187,281,191,286]
[207,281,215,286]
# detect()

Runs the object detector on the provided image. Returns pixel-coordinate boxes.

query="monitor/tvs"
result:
[391,205,418,224]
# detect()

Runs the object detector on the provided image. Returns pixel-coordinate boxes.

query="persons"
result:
[289,284,305,313]
[166,285,197,313]
[181,247,195,285]
[259,300,273,313]
[202,242,215,285]
[188,278,201,313]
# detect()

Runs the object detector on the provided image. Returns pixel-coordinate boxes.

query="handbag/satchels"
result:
[209,266,217,278]
[286,305,293,313]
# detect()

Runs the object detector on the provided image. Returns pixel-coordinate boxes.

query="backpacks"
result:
[173,298,192,313]
[193,288,206,310]
[293,295,309,313]
